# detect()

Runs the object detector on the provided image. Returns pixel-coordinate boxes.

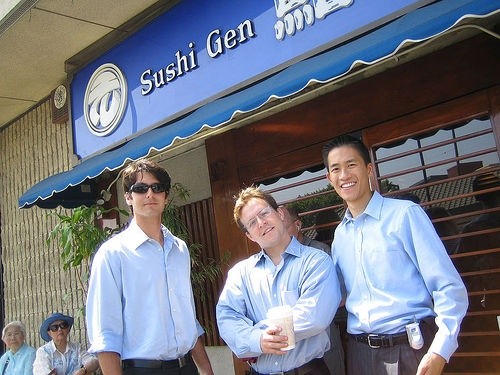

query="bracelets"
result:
[82,365,88,374]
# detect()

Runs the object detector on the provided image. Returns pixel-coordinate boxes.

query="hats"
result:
[40,313,74,342]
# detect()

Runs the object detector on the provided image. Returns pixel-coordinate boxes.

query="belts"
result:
[123,351,190,369]
[250,358,323,375]
[348,319,433,348]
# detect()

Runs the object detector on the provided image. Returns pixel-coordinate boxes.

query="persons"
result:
[86,160,215,375]
[0,321,37,375]
[282,207,346,307]
[33,313,101,375]
[322,133,469,375]
[216,187,341,375]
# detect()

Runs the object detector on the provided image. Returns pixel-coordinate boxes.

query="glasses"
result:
[128,183,169,199]
[244,205,273,228]
[47,321,69,332]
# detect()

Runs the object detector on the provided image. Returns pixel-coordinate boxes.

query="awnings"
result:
[18,1,500,212]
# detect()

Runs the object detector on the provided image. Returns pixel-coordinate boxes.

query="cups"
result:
[266,305,295,351]
